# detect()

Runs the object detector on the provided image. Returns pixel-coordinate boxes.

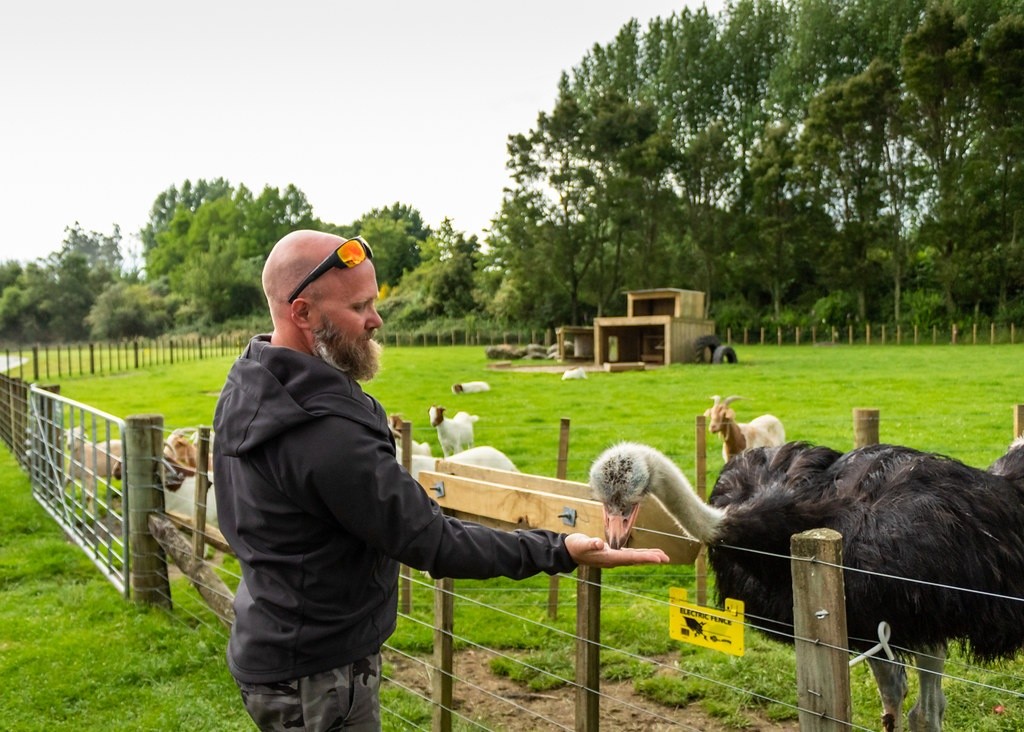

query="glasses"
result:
[285,235,373,303]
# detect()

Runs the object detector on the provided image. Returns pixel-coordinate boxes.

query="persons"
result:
[213,230,669,732]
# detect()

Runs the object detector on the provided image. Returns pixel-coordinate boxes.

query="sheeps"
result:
[60,428,123,500]
[428,406,478,458]
[453,381,490,394]
[162,429,226,562]
[391,415,521,476]
[562,364,587,380]
[705,394,787,463]
